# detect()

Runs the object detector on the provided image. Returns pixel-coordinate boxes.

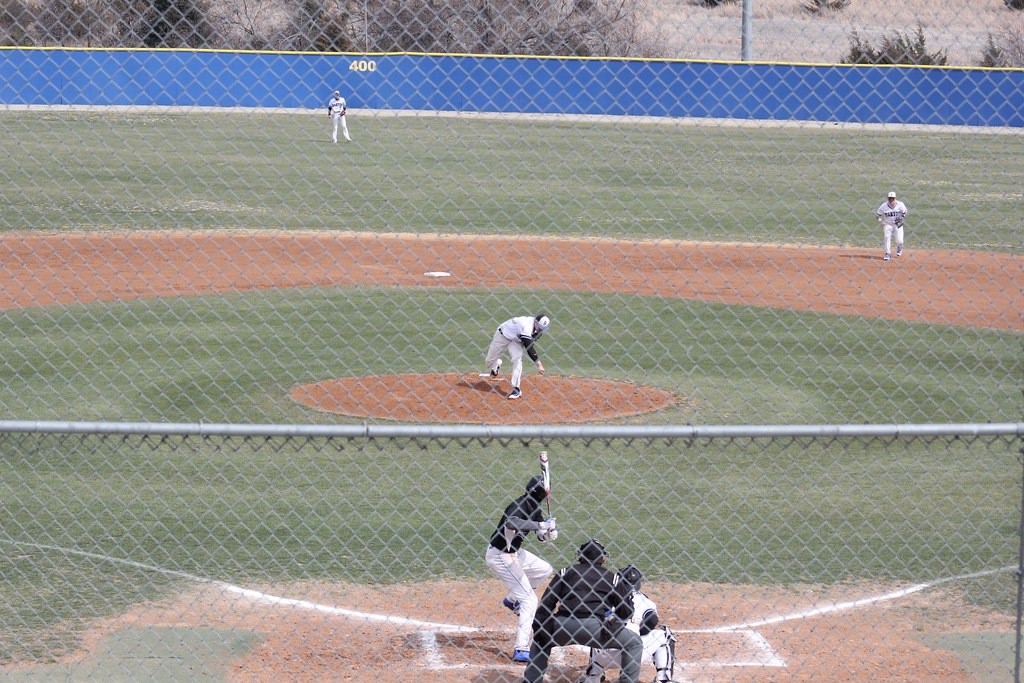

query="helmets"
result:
[526,475,551,502]
[617,565,643,590]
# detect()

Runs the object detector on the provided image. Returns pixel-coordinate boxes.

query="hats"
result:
[887,192,897,197]
[535,314,552,331]
[579,539,606,563]
[335,90,340,94]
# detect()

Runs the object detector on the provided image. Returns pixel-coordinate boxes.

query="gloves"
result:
[544,530,557,541]
[539,518,556,530]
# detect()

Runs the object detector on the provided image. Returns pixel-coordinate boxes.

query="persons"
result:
[328,90,352,143]
[485,476,676,683]
[875,192,907,260]
[486,314,550,399]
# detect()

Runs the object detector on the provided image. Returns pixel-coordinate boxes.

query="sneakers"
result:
[513,650,529,661]
[347,138,352,142]
[503,597,520,616]
[897,247,902,256]
[883,253,891,260]
[490,358,502,376]
[508,388,523,399]
[332,140,337,143]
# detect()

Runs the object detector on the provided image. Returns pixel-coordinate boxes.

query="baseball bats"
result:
[539,451,552,519]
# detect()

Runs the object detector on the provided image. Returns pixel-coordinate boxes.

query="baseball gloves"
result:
[894,217,904,228]
